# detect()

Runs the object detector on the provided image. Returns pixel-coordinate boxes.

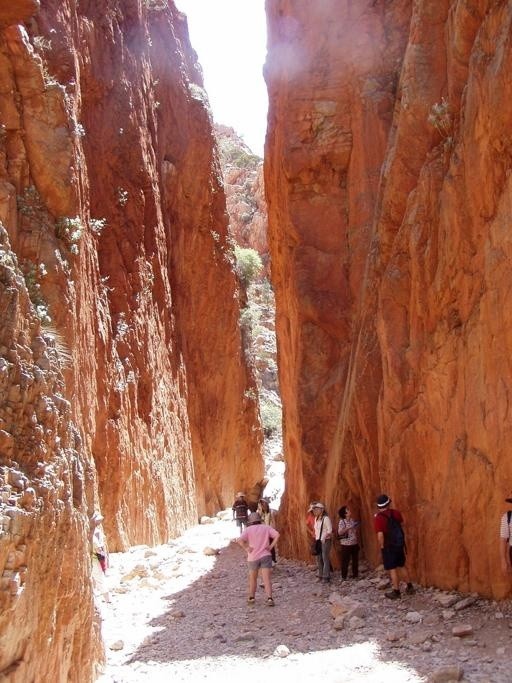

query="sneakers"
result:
[384,589,401,599]
[340,577,361,582]
[404,582,415,595]
[315,577,329,584]
[247,596,274,606]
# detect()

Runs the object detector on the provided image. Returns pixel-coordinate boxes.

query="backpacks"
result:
[379,509,405,549]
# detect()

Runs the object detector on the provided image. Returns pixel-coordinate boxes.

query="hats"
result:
[94,511,105,520]
[505,498,511,503]
[248,512,264,524]
[375,494,392,509]
[236,491,245,498]
[306,502,325,513]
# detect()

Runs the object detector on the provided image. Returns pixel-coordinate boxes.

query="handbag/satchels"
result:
[310,538,322,556]
[337,530,348,540]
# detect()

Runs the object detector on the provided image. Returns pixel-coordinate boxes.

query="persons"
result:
[499,490,512,575]
[304,501,334,574]
[236,512,282,607]
[311,502,333,584]
[258,499,277,563]
[337,506,362,580]
[90,511,109,577]
[373,494,417,600]
[232,492,249,532]
